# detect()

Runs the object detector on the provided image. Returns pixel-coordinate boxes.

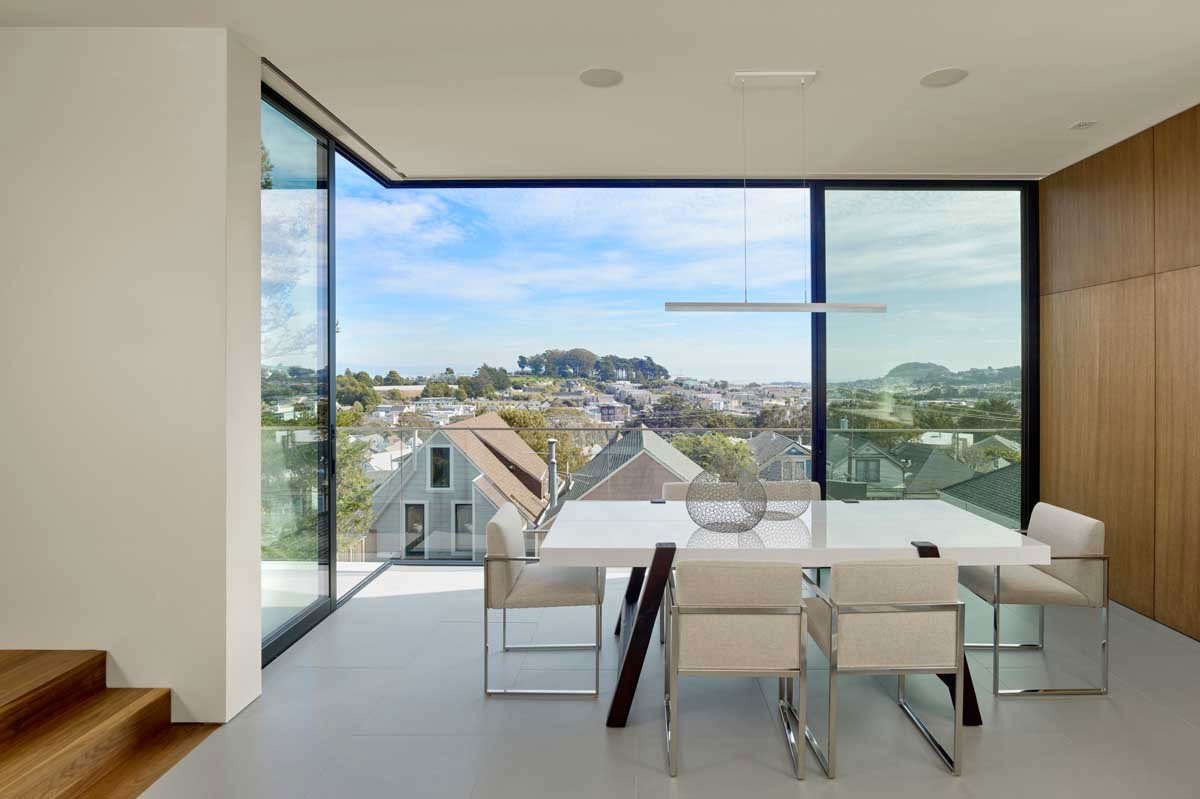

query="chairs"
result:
[953,500,1112,697]
[796,556,967,780]
[659,557,810,782]
[478,501,600,699]
[661,480,737,644]
[748,480,823,501]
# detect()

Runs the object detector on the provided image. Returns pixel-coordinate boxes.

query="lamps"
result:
[661,72,887,314]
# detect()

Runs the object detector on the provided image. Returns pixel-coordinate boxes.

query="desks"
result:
[542,494,1054,728]
